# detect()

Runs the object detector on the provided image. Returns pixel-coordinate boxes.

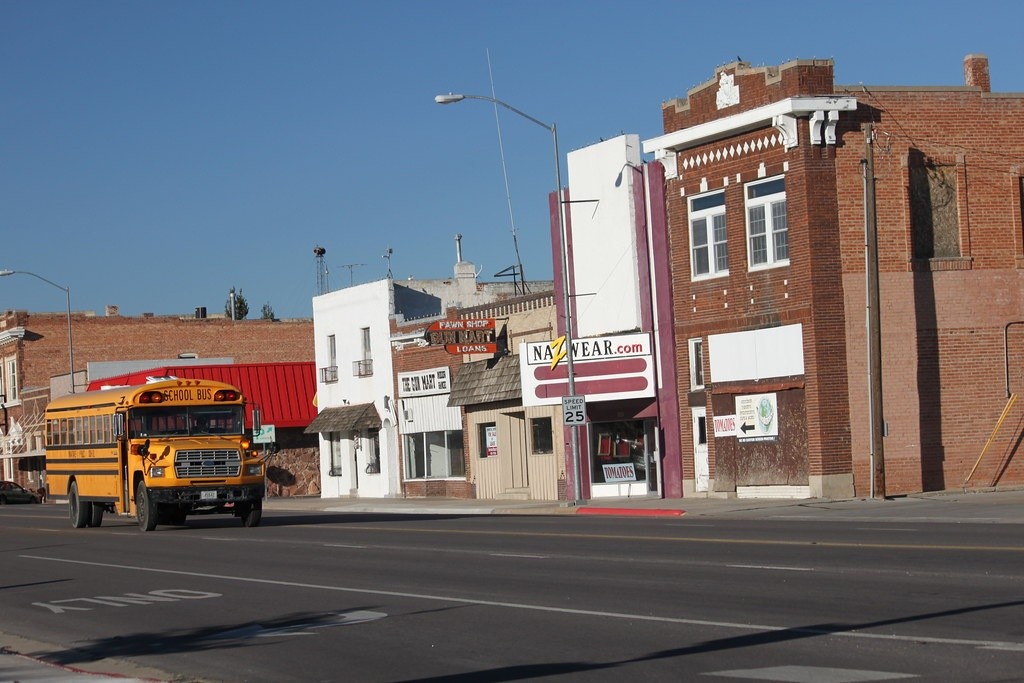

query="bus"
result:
[43,376,281,532]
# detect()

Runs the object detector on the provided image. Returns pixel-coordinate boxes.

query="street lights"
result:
[434,90,589,507]
[0,269,75,395]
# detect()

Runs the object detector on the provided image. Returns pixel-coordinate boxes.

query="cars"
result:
[0,480,42,506]
